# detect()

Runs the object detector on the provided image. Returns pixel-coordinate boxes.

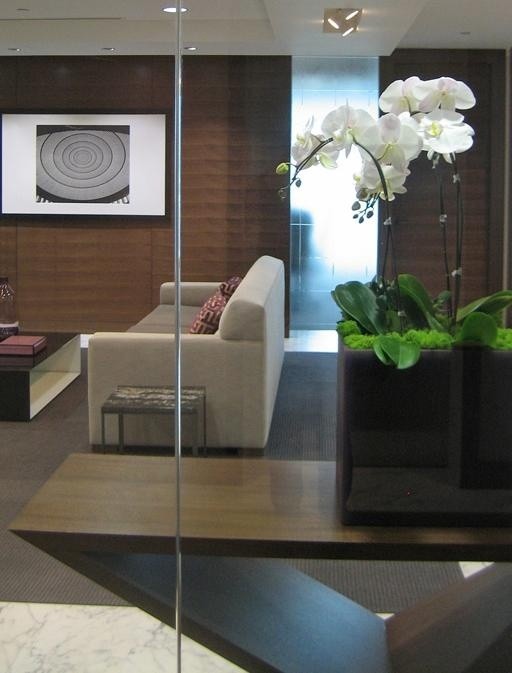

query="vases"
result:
[463,343,511,482]
[334,334,464,528]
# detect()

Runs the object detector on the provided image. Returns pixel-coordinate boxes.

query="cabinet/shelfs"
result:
[8,454,512,672]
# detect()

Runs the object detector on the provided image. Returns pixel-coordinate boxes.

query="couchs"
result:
[86,255,286,457]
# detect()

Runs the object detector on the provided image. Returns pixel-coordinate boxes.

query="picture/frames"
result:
[0,109,174,223]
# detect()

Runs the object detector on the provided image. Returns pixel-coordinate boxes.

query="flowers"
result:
[272,76,512,370]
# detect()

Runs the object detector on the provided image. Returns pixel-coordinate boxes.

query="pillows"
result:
[190,293,226,334]
[215,276,242,301]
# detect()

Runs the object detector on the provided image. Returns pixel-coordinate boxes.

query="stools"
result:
[101,385,206,456]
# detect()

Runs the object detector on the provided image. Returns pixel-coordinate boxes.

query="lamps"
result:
[323,8,362,37]
[0,277,20,340]
